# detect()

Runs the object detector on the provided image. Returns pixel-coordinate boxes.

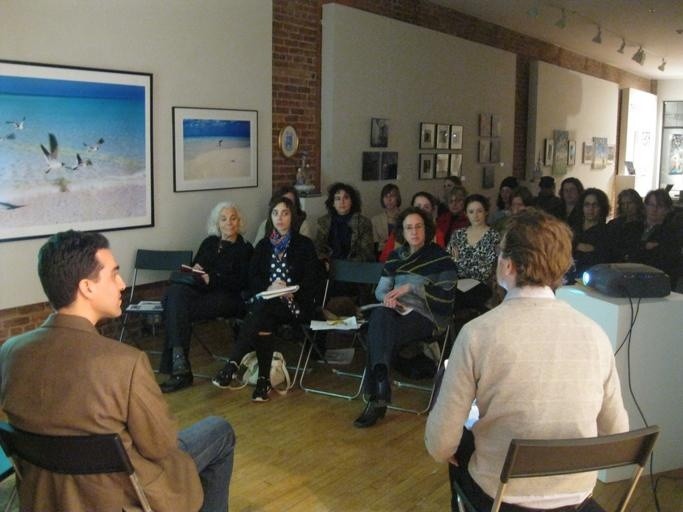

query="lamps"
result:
[524,0,668,72]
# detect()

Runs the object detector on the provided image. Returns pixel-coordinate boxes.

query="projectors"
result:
[582,262,672,299]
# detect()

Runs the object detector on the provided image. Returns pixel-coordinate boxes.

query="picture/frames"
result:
[0,59,154,241]
[418,122,464,179]
[583,137,614,170]
[173,106,258,193]
[544,130,576,176]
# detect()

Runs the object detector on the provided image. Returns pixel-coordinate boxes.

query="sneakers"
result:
[252,377,272,401]
[171,347,191,374]
[211,359,240,389]
[158,373,193,393]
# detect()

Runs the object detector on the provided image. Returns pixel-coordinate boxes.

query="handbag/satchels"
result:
[242,348,286,386]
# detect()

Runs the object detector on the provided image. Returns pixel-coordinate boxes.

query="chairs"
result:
[0,429,152,511]
[120,249,195,376]
[452,425,662,512]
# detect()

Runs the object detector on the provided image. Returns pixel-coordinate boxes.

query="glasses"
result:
[404,223,424,230]
[647,203,665,209]
[584,201,601,208]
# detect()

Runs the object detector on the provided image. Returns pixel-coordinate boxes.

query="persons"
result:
[425,207,630,510]
[0,231,235,512]
[306,181,373,360]
[353,205,457,429]
[212,195,321,403]
[372,175,682,292]
[159,200,253,392]
[252,186,318,250]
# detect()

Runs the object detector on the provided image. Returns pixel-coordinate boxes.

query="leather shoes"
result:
[354,401,387,427]
[370,378,392,406]
